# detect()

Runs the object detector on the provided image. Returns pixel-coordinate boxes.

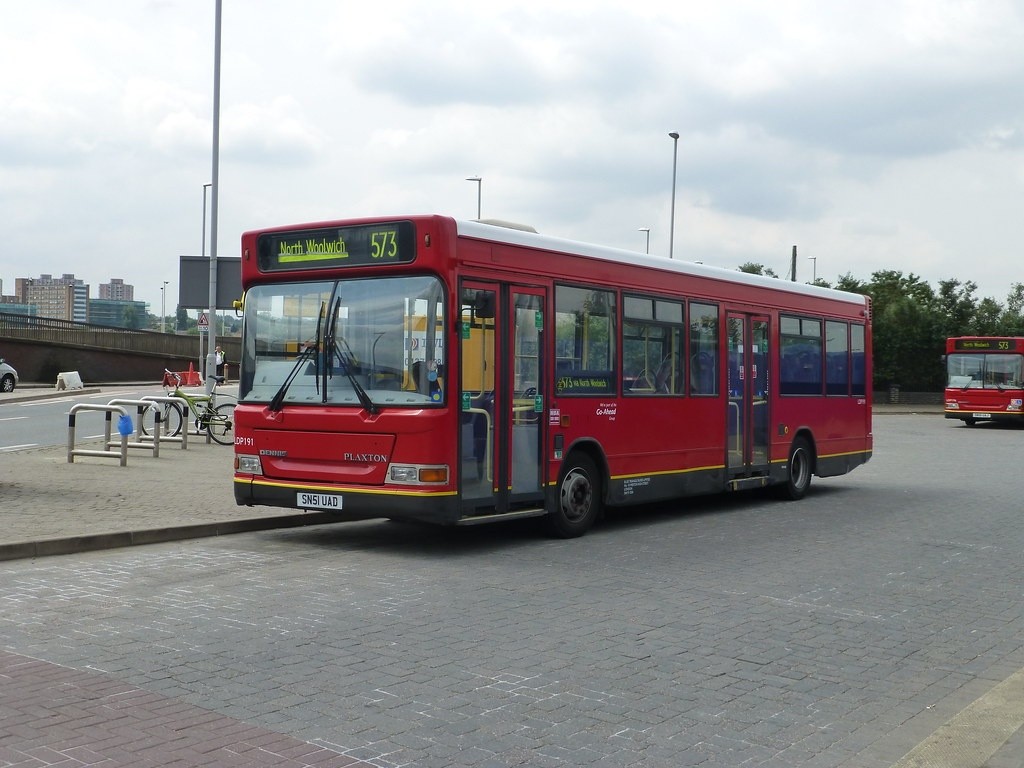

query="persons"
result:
[214,346,228,384]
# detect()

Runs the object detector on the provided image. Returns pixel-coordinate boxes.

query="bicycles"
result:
[141,368,238,444]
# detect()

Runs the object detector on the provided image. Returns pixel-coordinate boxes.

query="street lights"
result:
[638,229,650,254]
[808,257,816,286]
[198,183,212,384]
[667,131,680,258]
[466,177,481,218]
[68,281,73,330]
[27,276,33,326]
[160,281,170,333]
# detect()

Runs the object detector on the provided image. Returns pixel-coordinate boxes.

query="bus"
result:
[939,336,1024,426]
[233,215,875,537]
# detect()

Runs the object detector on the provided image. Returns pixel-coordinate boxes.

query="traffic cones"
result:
[184,362,198,386]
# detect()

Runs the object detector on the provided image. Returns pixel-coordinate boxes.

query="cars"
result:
[0,358,20,393]
